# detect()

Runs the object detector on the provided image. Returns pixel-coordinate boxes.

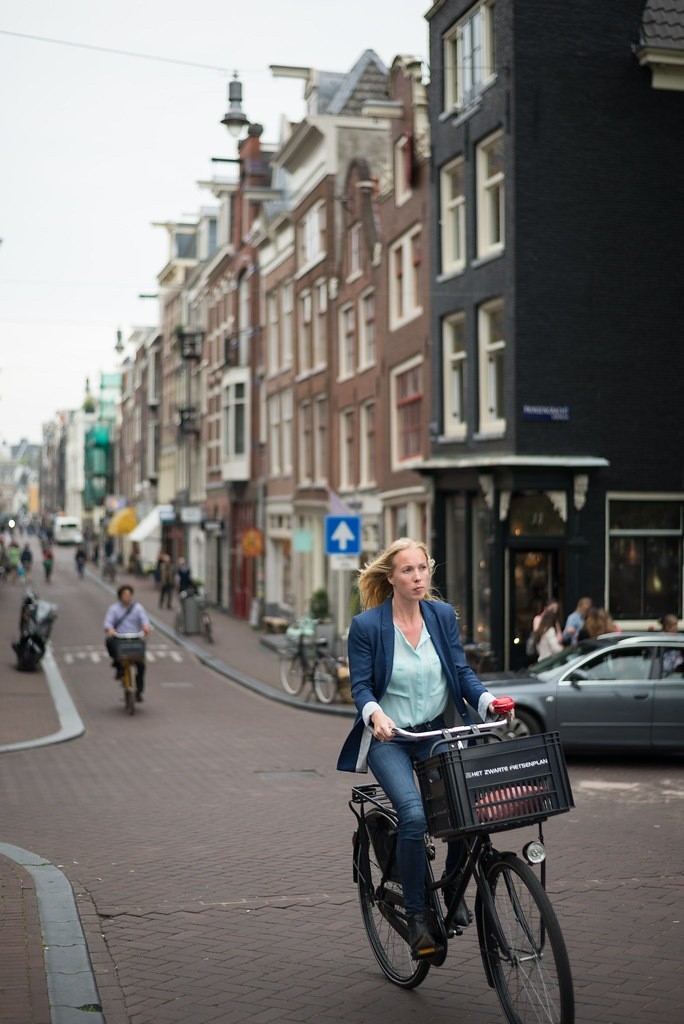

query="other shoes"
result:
[115,667,123,679]
[135,690,143,703]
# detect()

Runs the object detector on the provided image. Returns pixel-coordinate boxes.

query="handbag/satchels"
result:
[526,632,538,656]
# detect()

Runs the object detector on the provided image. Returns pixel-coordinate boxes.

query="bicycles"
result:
[113,630,146,716]
[345,698,578,1024]
[279,611,338,706]
[192,590,215,644]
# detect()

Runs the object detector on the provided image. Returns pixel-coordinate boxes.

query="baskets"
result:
[416,731,577,837]
[117,641,145,661]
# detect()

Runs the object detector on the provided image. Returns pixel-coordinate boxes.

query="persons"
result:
[157,553,191,609]
[335,538,515,950]
[533,593,678,678]
[103,584,151,703]
[0,516,88,582]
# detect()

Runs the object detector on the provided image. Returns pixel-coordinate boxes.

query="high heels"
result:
[440,869,471,927]
[407,913,435,950]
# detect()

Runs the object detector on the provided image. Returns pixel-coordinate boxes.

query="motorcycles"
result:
[10,591,60,673]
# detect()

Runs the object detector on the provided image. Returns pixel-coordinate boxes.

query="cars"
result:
[454,630,684,751]
